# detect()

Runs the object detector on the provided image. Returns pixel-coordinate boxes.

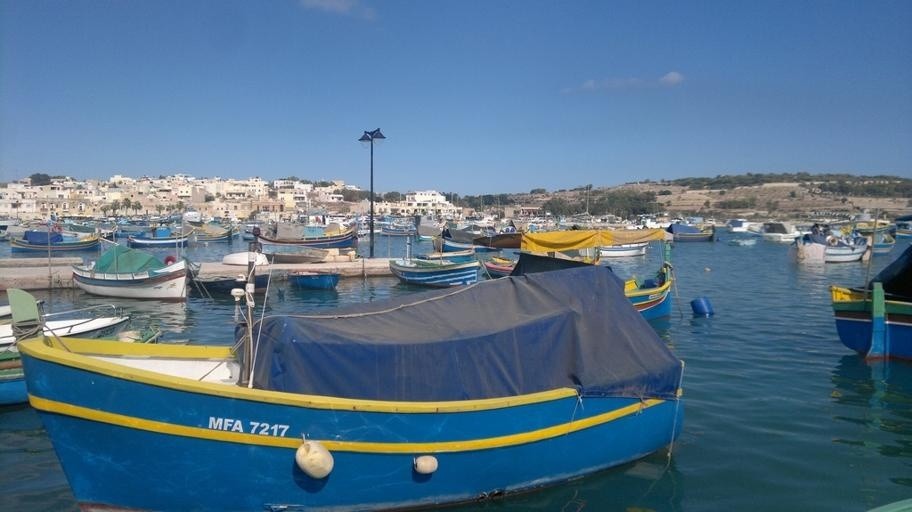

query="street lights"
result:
[359,126,388,258]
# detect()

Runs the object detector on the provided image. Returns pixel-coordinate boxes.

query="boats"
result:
[826,351,912,482]
[724,214,911,366]
